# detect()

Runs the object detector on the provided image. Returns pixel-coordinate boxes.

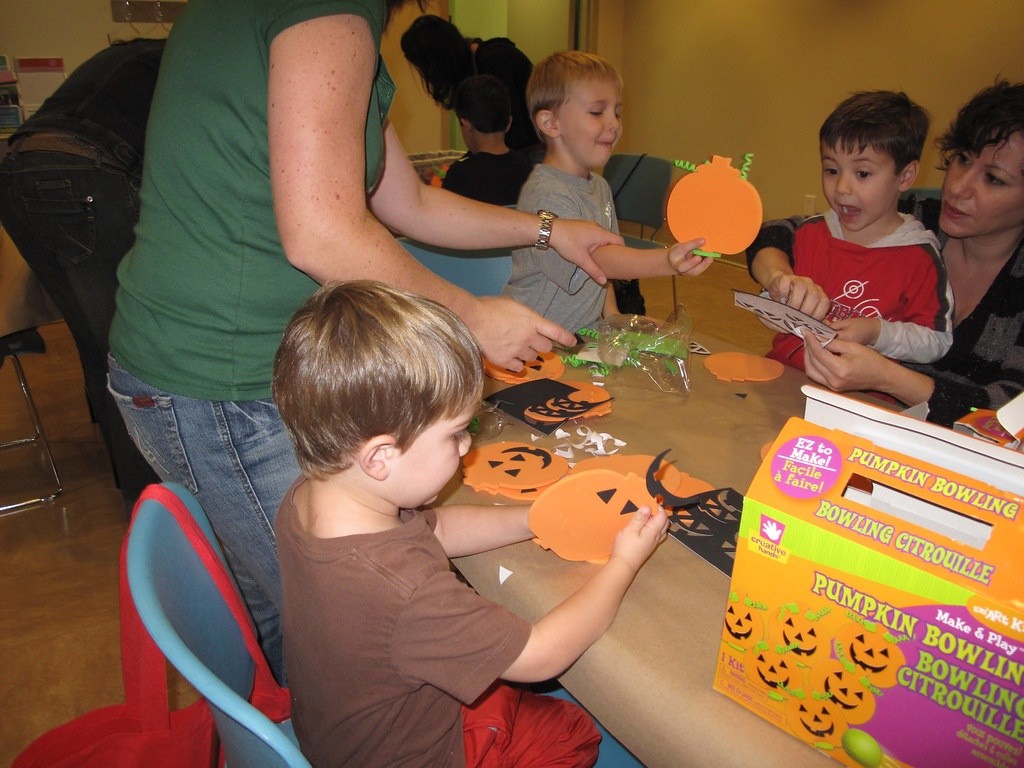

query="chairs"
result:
[127,483,645,768]
[603,154,678,319]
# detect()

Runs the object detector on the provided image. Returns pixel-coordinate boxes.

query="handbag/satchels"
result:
[8,483,291,768]
[612,279,646,315]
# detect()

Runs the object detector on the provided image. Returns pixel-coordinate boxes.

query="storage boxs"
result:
[712,384,1024,768]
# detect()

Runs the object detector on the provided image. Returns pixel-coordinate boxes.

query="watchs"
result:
[534,209,560,251]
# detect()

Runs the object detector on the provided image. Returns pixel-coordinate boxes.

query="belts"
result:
[4,136,125,168]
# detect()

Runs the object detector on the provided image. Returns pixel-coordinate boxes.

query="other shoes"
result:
[124,500,134,521]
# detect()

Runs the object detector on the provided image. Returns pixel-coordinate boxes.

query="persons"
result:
[1,36,168,520]
[401,14,713,335]
[106,0,627,692]
[745,68,1024,434]
[269,276,672,768]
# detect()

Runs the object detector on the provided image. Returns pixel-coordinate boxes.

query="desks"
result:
[434,321,905,768]
